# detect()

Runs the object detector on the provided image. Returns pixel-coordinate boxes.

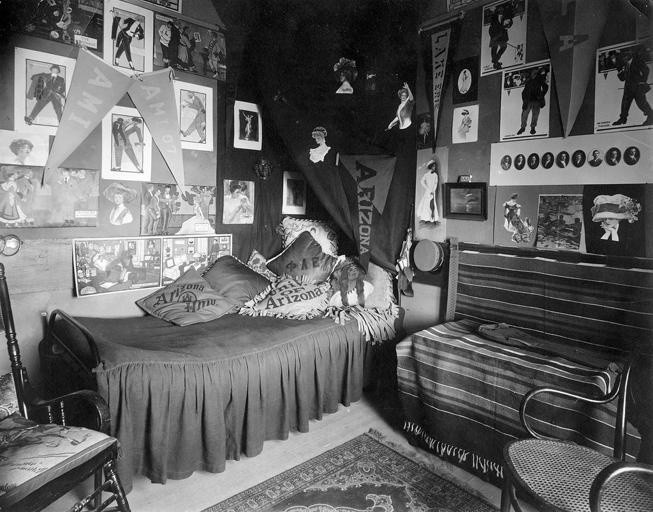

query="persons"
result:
[147,190,161,234]
[114,10,145,68]
[209,238,220,266]
[110,115,144,173]
[177,25,197,72]
[502,193,534,244]
[590,150,602,167]
[23,65,66,125]
[207,30,223,78]
[103,182,138,226]
[334,57,357,94]
[529,154,539,169]
[175,196,216,236]
[543,153,554,168]
[181,91,207,145]
[573,152,584,167]
[565,218,582,242]
[626,149,638,163]
[309,126,332,163]
[488,5,511,71]
[612,49,653,126]
[0,172,28,224]
[454,109,473,144]
[590,193,643,242]
[77,267,97,296]
[417,160,441,225]
[542,215,551,228]
[9,139,34,167]
[158,21,175,64]
[608,149,618,165]
[515,154,525,170]
[159,186,174,231]
[502,156,511,170]
[384,82,413,131]
[551,215,565,231]
[517,67,552,135]
[241,112,254,141]
[558,152,569,168]
[141,185,154,235]
[460,70,471,92]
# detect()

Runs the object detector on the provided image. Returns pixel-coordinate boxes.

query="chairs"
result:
[0,266,130,512]
[498,361,652,512]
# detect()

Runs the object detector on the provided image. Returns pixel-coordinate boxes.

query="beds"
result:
[40,216,405,496]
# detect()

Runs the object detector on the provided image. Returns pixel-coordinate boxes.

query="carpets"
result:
[200,427,500,511]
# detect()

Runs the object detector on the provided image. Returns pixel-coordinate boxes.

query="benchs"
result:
[396,237,653,498]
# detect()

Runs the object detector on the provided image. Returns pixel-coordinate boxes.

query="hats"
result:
[102,182,137,204]
[426,159,435,166]
[591,193,642,221]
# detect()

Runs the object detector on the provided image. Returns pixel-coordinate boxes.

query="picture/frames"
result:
[443,182,489,221]
[140,182,217,236]
[173,80,213,152]
[416,113,434,149]
[102,105,152,182]
[0,166,100,229]
[234,100,262,150]
[453,55,479,105]
[103,0,154,76]
[282,171,307,215]
[14,47,77,135]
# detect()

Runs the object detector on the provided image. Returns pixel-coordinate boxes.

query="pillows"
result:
[328,255,400,346]
[279,216,339,257]
[239,272,333,320]
[246,249,277,282]
[266,231,341,286]
[202,255,273,307]
[135,269,237,326]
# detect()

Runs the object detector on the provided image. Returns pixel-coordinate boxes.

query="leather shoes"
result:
[517,129,524,134]
[643,114,653,125]
[611,119,627,125]
[530,127,536,133]
